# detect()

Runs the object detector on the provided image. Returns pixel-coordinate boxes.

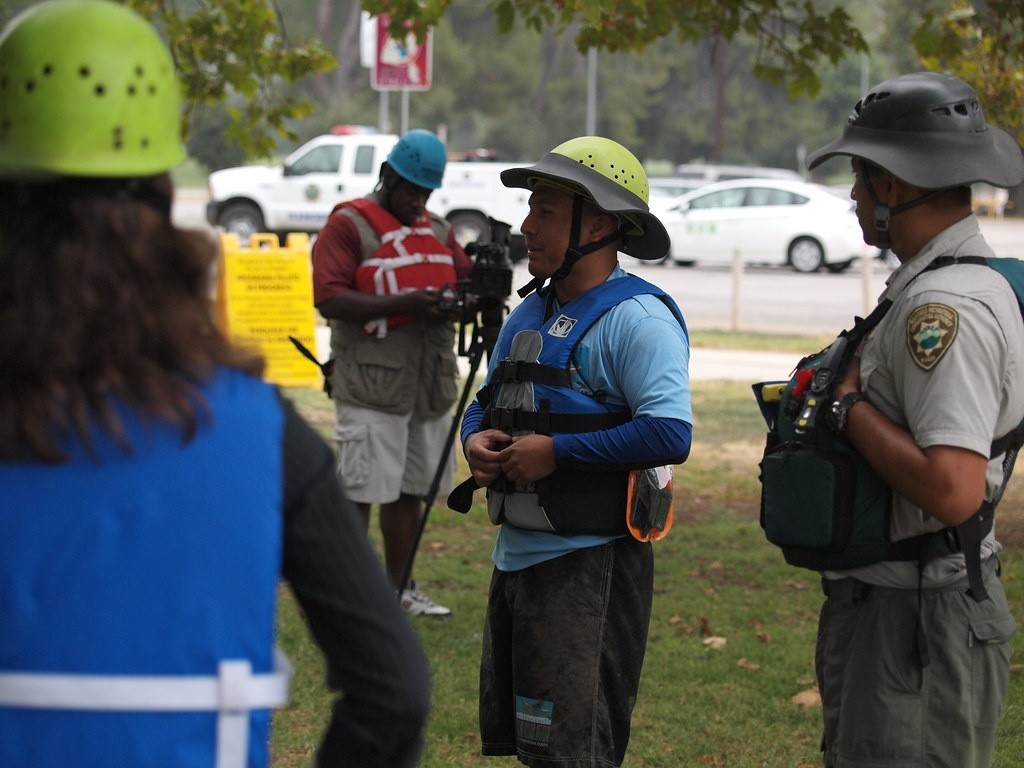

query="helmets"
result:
[496,136,672,261]
[805,70,1023,189]
[387,129,447,189]
[0,1,190,180]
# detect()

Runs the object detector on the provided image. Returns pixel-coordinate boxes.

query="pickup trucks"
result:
[205,123,540,262]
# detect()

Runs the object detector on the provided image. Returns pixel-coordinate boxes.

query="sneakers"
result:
[393,580,452,616]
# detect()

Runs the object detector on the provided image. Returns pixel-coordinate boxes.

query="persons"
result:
[460,135,693,768]
[0,0,432,768]
[311,129,483,616]
[806,70,1024,768]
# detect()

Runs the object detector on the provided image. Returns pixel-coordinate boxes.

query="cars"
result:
[645,179,880,274]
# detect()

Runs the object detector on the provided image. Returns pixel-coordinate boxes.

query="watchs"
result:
[824,391,865,433]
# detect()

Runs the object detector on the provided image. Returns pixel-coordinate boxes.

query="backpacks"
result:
[758,251,974,573]
